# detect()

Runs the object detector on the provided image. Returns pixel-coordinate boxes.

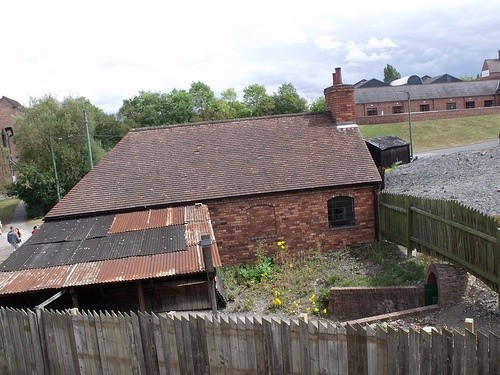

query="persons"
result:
[0,220,3,236]
[7,226,22,251]
[14,228,21,239]
[32,225,39,235]
